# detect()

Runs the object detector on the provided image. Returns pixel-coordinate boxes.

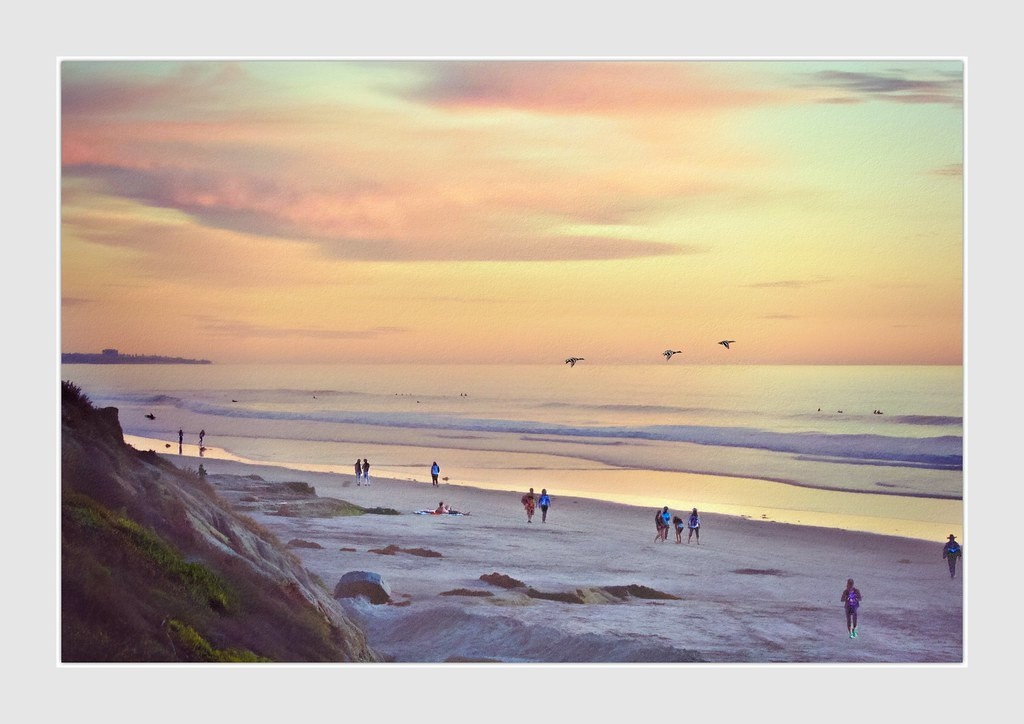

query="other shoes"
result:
[849,629,857,638]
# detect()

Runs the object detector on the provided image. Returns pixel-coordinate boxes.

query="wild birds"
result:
[232,400,238,402]
[874,410,883,414]
[718,340,736,349]
[838,410,843,413]
[313,396,318,399]
[144,413,156,420]
[564,357,584,368]
[395,393,411,396]
[417,401,419,403]
[663,350,682,361]
[817,408,822,412]
[460,393,467,397]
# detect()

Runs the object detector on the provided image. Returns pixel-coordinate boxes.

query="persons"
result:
[654,506,671,544]
[199,464,205,477]
[688,508,700,545]
[199,429,205,444]
[431,461,440,487]
[673,516,684,543]
[436,502,470,515]
[355,459,371,486]
[179,430,183,441]
[525,488,552,523]
[943,534,961,578]
[841,578,862,639]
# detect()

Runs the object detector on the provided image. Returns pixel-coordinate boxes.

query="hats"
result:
[947,534,956,539]
